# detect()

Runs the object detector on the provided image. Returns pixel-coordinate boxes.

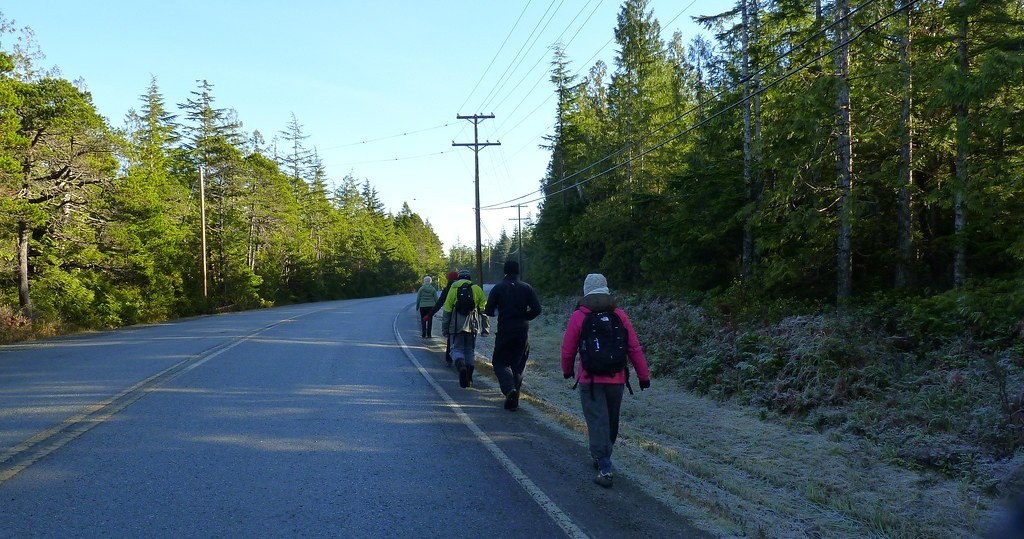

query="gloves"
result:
[424,315,429,321]
[639,380,651,392]
[563,371,575,379]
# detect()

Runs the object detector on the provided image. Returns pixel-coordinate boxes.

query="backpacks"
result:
[575,306,628,378]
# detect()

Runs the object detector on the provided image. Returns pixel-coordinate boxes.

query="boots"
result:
[456,359,467,388]
[467,365,474,385]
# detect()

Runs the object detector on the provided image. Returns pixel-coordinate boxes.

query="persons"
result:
[484,260,543,412]
[416,276,438,338]
[560,274,652,489]
[423,272,458,367]
[441,269,487,388]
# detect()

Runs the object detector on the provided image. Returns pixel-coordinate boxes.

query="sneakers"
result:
[593,470,614,488]
[587,456,600,468]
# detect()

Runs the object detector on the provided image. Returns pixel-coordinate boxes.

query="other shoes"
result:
[504,386,519,412]
[445,362,451,367]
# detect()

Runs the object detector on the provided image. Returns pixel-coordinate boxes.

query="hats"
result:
[504,261,519,275]
[449,272,458,280]
[424,277,432,283]
[584,274,607,295]
[458,270,470,277]
